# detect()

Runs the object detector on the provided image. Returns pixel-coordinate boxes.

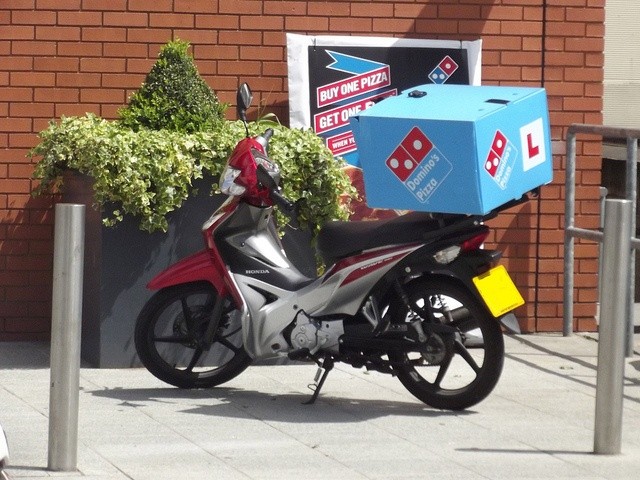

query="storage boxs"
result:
[348,83,554,216]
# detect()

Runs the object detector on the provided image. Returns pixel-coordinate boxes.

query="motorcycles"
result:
[133,81,530,412]
